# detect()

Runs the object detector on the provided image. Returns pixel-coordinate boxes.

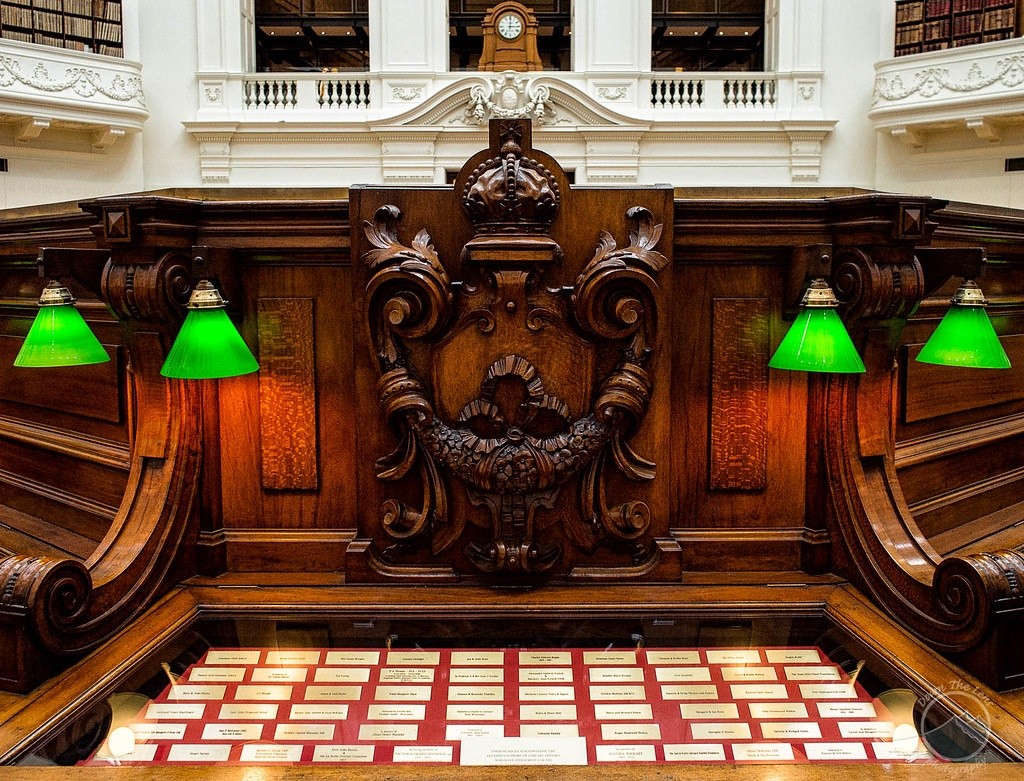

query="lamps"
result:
[13,280,111,367]
[914,276,1012,369]
[160,280,259,380]
[766,278,868,374]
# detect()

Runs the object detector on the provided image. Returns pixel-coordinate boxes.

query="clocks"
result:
[498,16,522,38]
